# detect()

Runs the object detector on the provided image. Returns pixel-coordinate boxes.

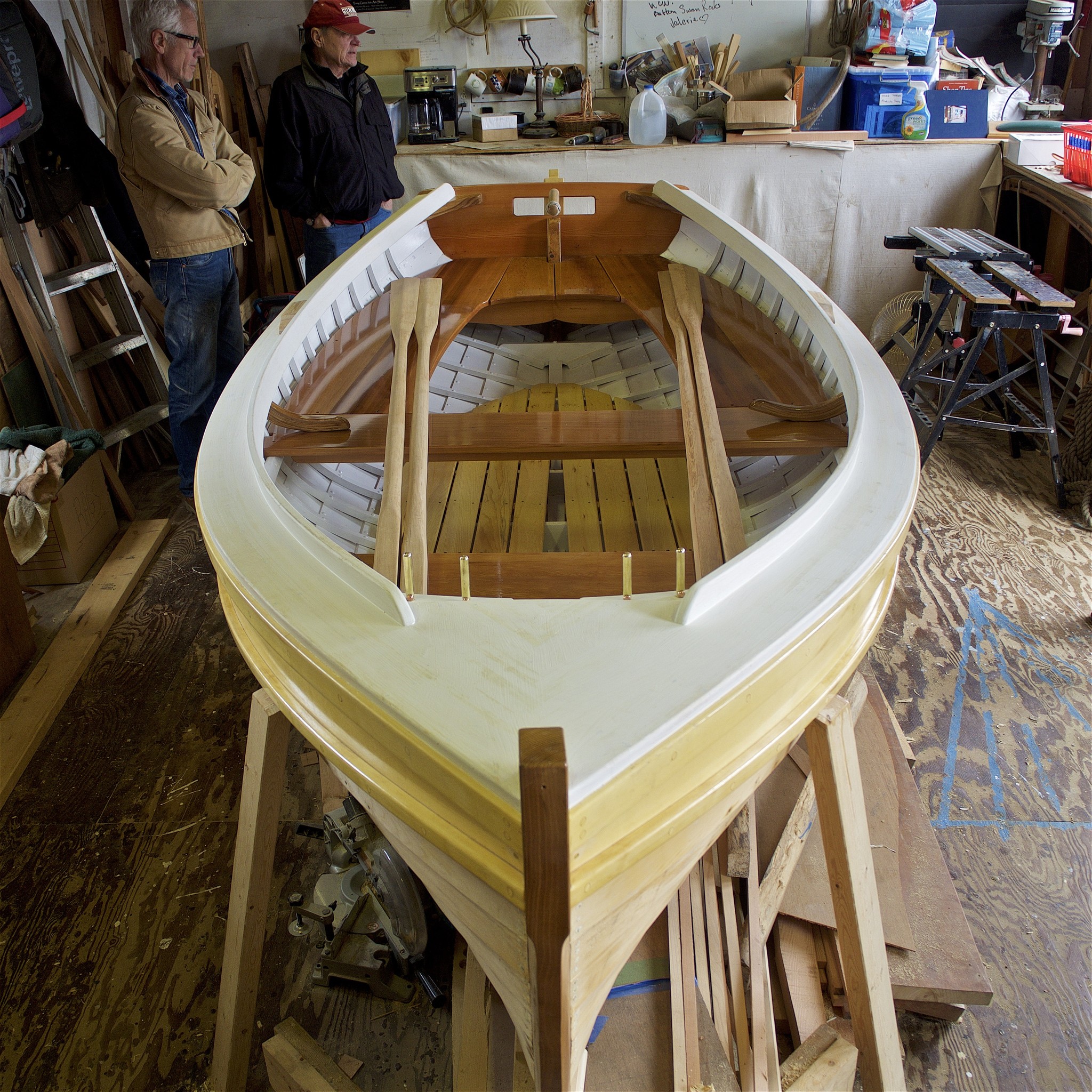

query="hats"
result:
[302,0,376,36]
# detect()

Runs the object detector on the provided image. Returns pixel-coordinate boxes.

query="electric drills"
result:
[565,126,606,146]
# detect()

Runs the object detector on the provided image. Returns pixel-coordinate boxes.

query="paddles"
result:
[373,277,444,595]
[656,262,746,582]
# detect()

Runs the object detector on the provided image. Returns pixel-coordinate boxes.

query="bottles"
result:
[628,84,667,146]
[691,89,716,110]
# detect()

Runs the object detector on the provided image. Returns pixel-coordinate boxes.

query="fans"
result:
[870,289,952,405]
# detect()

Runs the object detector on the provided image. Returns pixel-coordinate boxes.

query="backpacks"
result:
[0,0,44,148]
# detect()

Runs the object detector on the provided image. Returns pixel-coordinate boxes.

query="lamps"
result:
[485,0,559,139]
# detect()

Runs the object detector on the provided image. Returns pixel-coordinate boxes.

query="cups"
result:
[524,69,546,94]
[544,67,565,96]
[598,119,625,136]
[487,69,508,94]
[508,112,525,124]
[608,69,625,90]
[505,67,526,96]
[463,70,488,97]
[564,66,584,95]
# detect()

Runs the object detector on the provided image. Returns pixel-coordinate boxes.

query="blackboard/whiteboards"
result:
[621,1,812,89]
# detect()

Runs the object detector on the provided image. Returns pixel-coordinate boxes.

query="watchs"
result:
[305,212,321,226]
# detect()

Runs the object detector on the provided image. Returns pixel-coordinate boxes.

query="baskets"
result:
[1061,122,1092,187]
[555,78,622,138]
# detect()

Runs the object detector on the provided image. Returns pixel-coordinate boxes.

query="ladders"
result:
[0,173,170,523]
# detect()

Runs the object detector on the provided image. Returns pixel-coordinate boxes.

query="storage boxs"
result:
[472,112,519,142]
[1007,121,1092,188]
[708,67,803,130]
[842,63,934,138]
[382,96,407,145]
[785,60,843,130]
[13,452,118,585]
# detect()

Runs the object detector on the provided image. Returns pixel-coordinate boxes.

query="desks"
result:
[1001,155,1092,431]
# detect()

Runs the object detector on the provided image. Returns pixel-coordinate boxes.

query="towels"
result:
[0,423,108,566]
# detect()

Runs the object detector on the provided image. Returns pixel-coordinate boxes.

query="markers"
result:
[617,57,625,70]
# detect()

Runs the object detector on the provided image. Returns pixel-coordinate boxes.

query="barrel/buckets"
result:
[686,86,725,100]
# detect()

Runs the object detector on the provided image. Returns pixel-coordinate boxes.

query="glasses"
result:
[164,30,200,48]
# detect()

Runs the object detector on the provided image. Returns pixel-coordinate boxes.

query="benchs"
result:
[266,252,848,464]
[352,548,693,598]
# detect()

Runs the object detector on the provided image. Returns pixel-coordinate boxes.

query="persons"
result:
[263,0,405,286]
[111,0,260,520]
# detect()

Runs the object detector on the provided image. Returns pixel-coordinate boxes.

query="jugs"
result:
[407,94,444,131]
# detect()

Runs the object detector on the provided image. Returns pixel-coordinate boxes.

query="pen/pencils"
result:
[594,111,603,121]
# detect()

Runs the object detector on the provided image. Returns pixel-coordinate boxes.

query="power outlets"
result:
[457,87,471,112]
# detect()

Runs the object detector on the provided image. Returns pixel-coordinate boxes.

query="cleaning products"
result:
[900,79,930,141]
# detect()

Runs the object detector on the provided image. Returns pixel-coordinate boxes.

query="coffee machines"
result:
[403,65,460,145]
